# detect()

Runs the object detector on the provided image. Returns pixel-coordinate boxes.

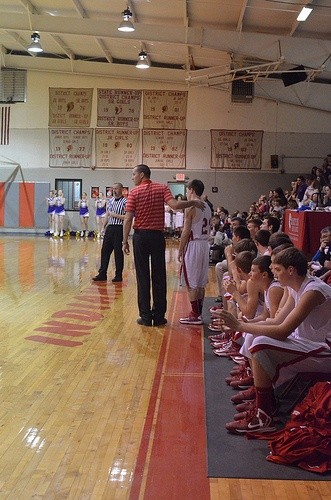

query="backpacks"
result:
[245,381,331,475]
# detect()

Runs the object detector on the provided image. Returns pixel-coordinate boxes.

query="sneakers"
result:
[179,314,203,324]
[208,303,278,434]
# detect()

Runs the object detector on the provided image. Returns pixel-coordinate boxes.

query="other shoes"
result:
[112,277,123,282]
[153,318,167,326]
[92,274,107,281]
[137,318,152,326]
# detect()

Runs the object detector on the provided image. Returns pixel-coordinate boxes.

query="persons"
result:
[93,183,127,282]
[48,190,58,235]
[177,180,211,325]
[164,194,184,239]
[95,192,107,238]
[122,164,206,327]
[207,153,331,434]
[54,190,65,237]
[78,193,89,237]
[203,195,213,211]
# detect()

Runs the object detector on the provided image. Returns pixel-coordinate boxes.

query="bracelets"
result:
[122,242,128,244]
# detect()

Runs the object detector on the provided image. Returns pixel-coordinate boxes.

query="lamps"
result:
[116,2,136,33]
[135,46,150,70]
[26,33,43,53]
[295,2,314,23]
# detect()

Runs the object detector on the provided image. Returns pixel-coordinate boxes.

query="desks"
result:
[284,208,331,258]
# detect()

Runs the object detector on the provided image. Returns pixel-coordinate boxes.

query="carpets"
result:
[199,296,331,481]
